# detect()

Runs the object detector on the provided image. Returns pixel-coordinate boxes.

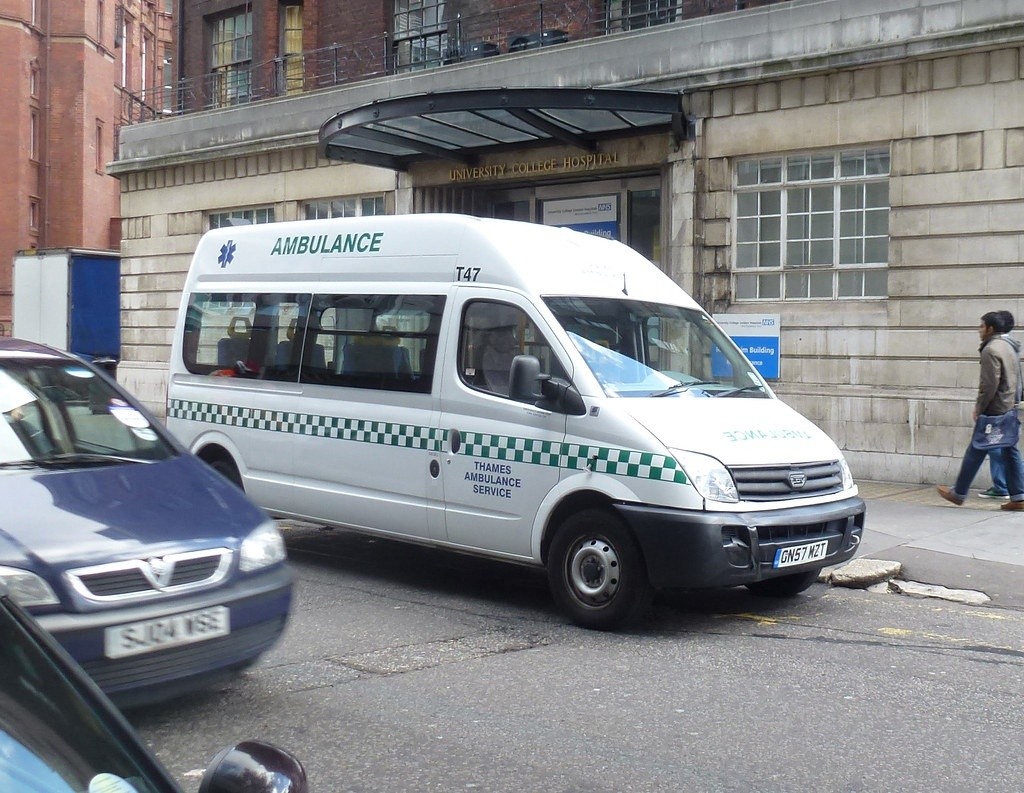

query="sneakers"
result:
[1001,502,1024,510]
[937,486,963,506]
[978,487,1010,499]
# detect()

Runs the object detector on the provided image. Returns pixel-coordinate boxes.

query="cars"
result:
[0,335,296,712]
[1,584,306,793]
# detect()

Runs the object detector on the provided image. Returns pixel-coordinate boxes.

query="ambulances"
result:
[166,211,866,631]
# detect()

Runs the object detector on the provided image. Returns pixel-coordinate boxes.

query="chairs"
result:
[277,318,415,376]
[217,316,252,369]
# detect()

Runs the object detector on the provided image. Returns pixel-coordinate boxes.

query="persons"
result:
[937,310,1024,511]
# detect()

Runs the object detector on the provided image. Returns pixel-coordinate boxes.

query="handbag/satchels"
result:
[972,409,1019,450]
